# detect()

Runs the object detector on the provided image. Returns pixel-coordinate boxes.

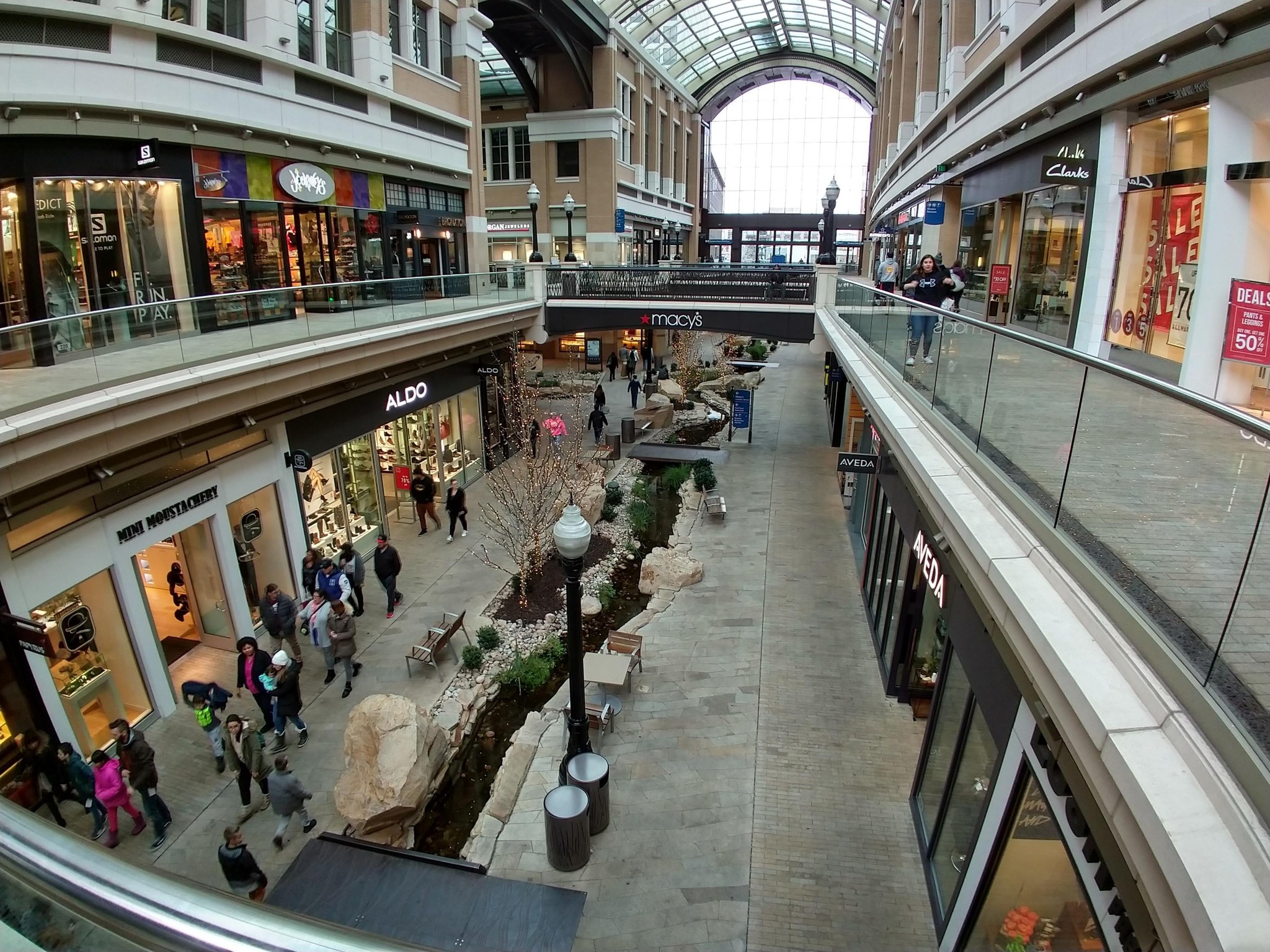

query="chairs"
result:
[596,445,615,467]
[405,609,472,682]
[561,628,643,756]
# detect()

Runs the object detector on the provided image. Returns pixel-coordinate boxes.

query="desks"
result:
[583,652,631,716]
[583,450,610,468]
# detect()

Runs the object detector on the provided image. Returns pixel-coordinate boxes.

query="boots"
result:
[259,712,274,734]
[105,811,146,848]
[951,308,960,322]
[324,668,337,684]
[240,793,269,821]
[222,738,226,752]
[215,755,226,773]
[269,722,310,753]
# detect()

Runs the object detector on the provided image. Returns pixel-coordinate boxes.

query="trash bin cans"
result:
[567,752,610,836]
[544,785,591,872]
[562,273,576,296]
[654,356,662,371]
[621,417,635,443]
[646,383,656,402]
[605,432,620,460]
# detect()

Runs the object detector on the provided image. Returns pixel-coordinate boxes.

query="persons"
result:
[606,352,618,382]
[594,384,606,413]
[640,344,654,371]
[221,713,273,822]
[905,251,967,331]
[900,254,956,365]
[302,548,322,598]
[703,255,714,268]
[218,826,268,902]
[338,542,365,616]
[445,478,468,542]
[523,413,541,459]
[542,412,567,460]
[260,583,303,671]
[258,650,309,754]
[325,600,362,698]
[627,375,642,410]
[588,404,608,447]
[297,589,341,684]
[877,252,899,315]
[315,558,352,605]
[767,264,787,300]
[181,681,233,773]
[373,534,404,618]
[236,637,272,733]
[619,343,640,381]
[268,755,317,850]
[20,719,173,852]
[410,467,441,536]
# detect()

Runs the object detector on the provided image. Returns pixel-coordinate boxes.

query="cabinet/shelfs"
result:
[208,243,375,330]
[307,405,480,565]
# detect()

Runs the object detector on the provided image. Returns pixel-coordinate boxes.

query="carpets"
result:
[161,636,202,666]
[1013,781,1061,840]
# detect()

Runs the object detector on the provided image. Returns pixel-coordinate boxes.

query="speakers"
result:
[1205,23,1229,45]
[242,130,253,140]
[4,107,21,119]
[1041,106,1056,119]
[997,130,1007,141]
[319,145,331,154]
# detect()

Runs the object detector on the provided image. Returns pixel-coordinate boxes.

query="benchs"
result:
[635,419,654,439]
[700,484,727,527]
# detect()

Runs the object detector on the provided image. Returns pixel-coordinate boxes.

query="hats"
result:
[339,542,353,549]
[234,525,241,536]
[934,252,942,264]
[319,559,332,569]
[376,534,388,543]
[272,649,289,665]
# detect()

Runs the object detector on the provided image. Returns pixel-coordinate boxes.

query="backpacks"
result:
[255,730,265,748]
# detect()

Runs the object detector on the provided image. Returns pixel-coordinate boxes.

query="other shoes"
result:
[417,524,441,537]
[210,236,375,314]
[905,356,933,366]
[385,595,404,618]
[342,663,362,698]
[446,530,469,544]
[91,813,108,840]
[310,409,477,559]
[151,819,172,852]
[272,819,318,850]
[352,608,365,618]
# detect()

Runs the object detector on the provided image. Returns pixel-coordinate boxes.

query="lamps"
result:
[192,124,198,132]
[284,140,290,147]
[339,377,358,391]
[452,174,458,179]
[980,144,986,150]
[1158,54,1167,66]
[409,164,414,170]
[75,111,81,121]
[1020,122,1028,130]
[355,154,360,159]
[1075,92,1084,103]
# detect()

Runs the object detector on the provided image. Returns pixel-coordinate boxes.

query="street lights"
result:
[552,503,597,787]
[661,216,669,260]
[674,219,681,260]
[526,179,544,263]
[815,174,840,265]
[562,189,577,262]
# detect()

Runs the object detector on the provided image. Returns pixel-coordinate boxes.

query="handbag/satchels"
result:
[606,363,611,368]
[300,618,311,636]
[950,273,965,291]
